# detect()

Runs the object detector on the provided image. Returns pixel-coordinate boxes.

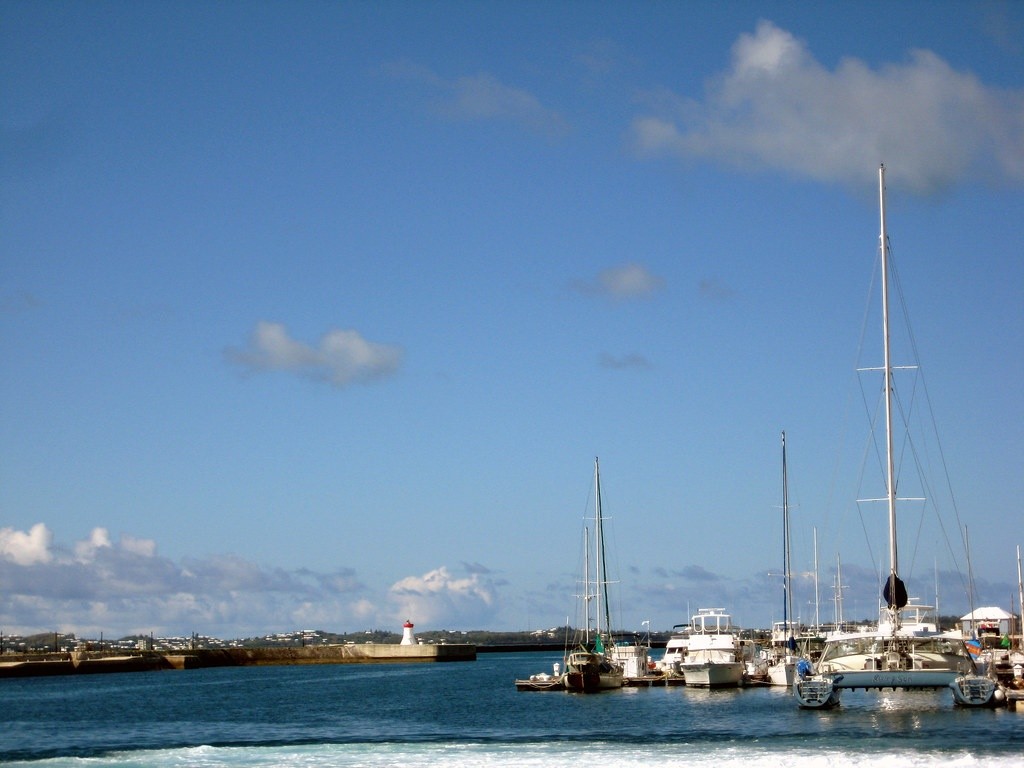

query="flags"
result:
[1000,634,1010,647]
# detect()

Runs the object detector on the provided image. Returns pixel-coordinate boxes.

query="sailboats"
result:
[513,160,1024,714]
